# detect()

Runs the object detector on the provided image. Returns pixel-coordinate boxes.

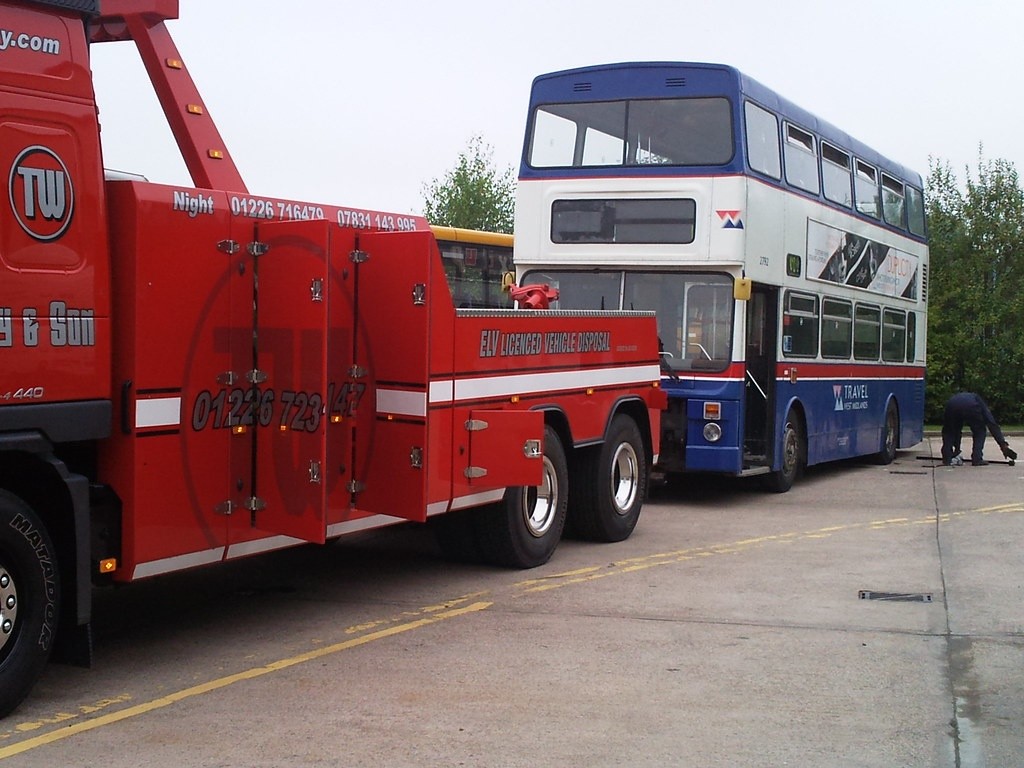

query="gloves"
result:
[954,444,961,454]
[1002,447,1017,460]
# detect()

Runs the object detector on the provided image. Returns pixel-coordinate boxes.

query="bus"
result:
[510,62,928,495]
[430,219,737,364]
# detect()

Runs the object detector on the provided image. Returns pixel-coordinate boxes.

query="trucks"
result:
[0,0,667,717]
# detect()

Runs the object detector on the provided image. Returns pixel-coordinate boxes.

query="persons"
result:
[941,392,1017,466]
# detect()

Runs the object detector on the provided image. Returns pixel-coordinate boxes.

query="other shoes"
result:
[971,458,989,466]
[942,458,951,465]
